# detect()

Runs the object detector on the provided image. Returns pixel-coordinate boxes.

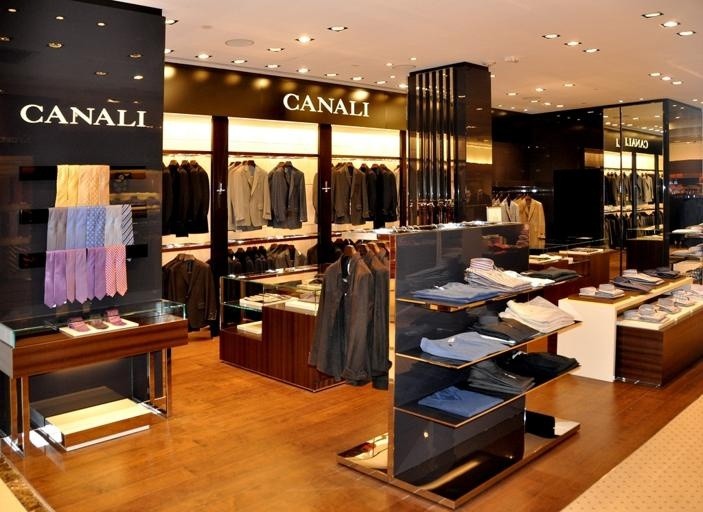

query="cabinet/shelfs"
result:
[605,153,663,244]
[557,272,702,385]
[162,151,213,338]
[228,154,315,324]
[671,184,703,279]
[330,155,398,262]
[337,229,578,489]
[23,168,149,289]
[530,249,617,296]
[493,189,554,247]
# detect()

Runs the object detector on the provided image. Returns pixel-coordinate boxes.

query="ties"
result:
[104,310,126,325]
[54,165,110,207]
[88,314,108,329]
[68,316,90,332]
[43,205,135,309]
